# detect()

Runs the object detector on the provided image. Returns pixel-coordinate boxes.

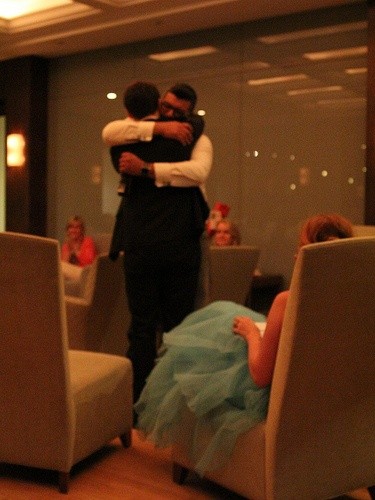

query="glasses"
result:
[161,102,185,117]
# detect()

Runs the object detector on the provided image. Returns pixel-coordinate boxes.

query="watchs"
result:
[141,163,151,178]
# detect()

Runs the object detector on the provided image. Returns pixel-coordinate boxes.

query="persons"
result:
[131,214,352,480]
[60,79,239,432]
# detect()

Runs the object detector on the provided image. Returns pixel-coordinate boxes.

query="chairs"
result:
[0,231,133,493]
[63,251,130,358]
[173,236,375,500]
[201,242,260,307]
